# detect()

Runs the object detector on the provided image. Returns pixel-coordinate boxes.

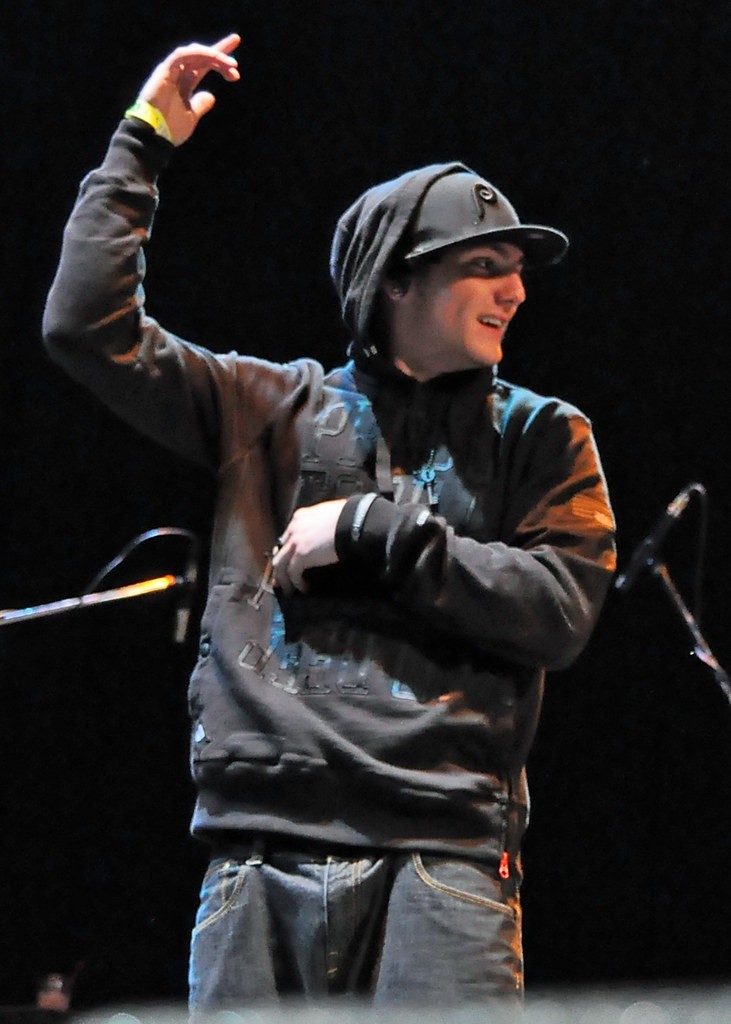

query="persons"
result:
[39,34,615,1013]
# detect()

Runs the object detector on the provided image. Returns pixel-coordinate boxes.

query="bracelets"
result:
[124,101,171,141]
[351,494,381,542]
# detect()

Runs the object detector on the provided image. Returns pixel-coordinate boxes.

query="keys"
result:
[420,468,438,506]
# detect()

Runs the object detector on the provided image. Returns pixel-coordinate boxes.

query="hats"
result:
[387,170,569,272]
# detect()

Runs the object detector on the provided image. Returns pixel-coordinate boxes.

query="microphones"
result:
[614,484,697,589]
[175,546,203,644]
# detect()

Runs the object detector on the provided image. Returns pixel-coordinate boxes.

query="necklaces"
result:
[420,447,434,469]
